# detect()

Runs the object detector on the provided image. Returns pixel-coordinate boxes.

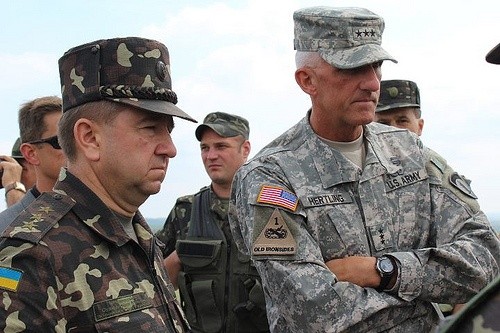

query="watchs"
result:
[374,255,397,294]
[4,181,26,201]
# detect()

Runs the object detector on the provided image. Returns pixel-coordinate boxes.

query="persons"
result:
[0,37,193,333]
[154,112,270,333]
[229,6,500,333]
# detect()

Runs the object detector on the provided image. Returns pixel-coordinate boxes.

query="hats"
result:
[375,80,420,112]
[11,138,23,158]
[59,37,197,122]
[195,112,250,142]
[293,6,398,67]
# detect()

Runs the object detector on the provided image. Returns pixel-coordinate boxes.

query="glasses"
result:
[27,136,61,149]
